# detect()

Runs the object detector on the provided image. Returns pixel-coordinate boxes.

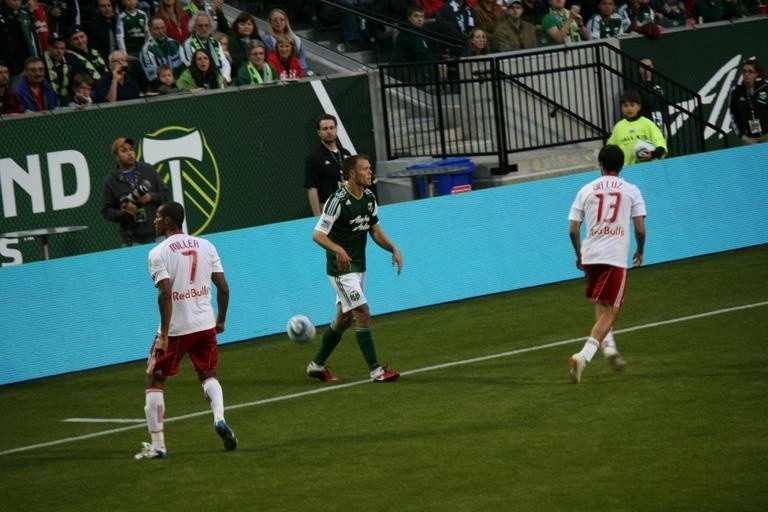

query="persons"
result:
[301,113,352,216]
[308,0,766,90]
[100,134,169,249]
[132,201,240,460]
[568,143,646,386]
[0,0,308,117]
[727,60,768,143]
[304,154,404,383]
[631,55,674,157]
[602,89,670,167]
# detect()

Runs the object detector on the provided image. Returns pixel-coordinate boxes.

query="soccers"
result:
[635,140,655,160]
[287,316,315,341]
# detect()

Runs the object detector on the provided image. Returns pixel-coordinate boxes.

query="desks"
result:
[0,225,89,260]
[387,166,469,199]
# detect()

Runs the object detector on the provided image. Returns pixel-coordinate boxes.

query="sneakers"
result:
[132,441,168,460]
[567,354,586,385]
[602,347,628,374]
[213,416,239,451]
[305,360,337,383]
[369,363,402,382]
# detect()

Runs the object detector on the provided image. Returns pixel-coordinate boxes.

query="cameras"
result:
[117,64,131,74]
[116,186,149,207]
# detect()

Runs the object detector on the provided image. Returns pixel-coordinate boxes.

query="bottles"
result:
[604,24,620,39]
[643,13,652,25]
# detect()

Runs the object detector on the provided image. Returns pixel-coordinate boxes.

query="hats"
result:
[506,0,524,8]
[67,24,87,38]
[47,32,68,45]
[111,136,134,154]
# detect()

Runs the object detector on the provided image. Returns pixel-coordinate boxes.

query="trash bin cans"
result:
[405,157,476,200]
